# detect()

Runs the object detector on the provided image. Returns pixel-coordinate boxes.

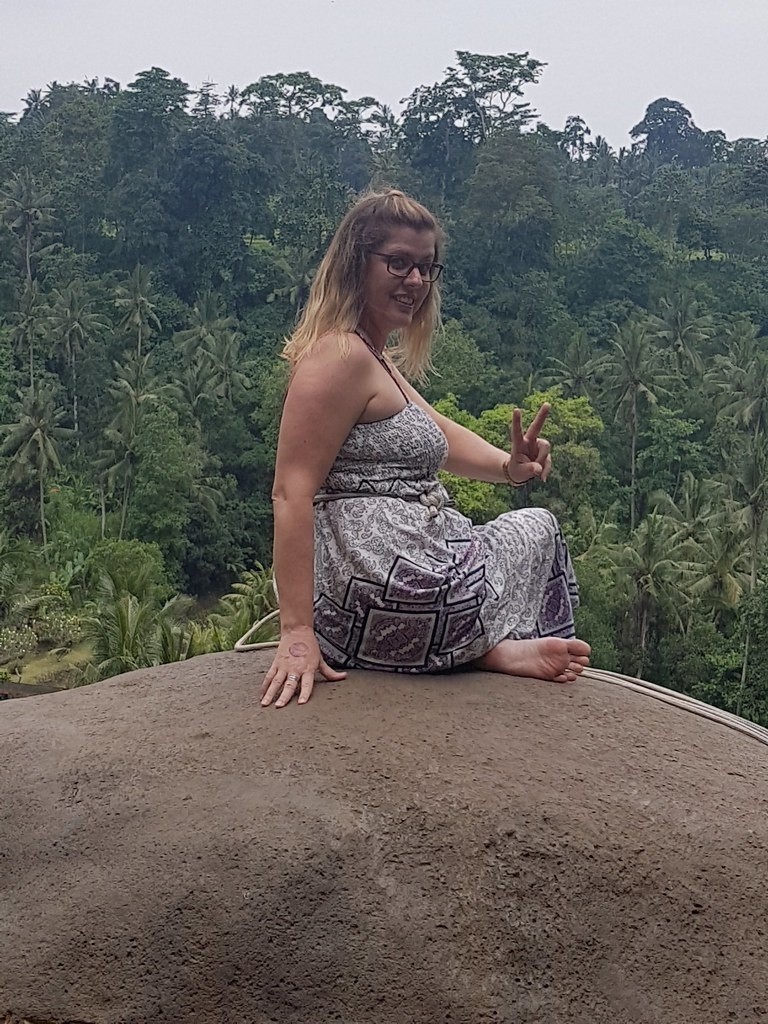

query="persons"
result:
[259,192,594,707]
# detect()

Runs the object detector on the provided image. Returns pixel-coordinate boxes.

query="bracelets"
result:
[503,457,534,486]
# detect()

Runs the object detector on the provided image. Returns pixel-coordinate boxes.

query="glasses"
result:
[368,250,445,282]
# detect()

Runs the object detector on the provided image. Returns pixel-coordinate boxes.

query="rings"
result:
[287,675,299,683]
